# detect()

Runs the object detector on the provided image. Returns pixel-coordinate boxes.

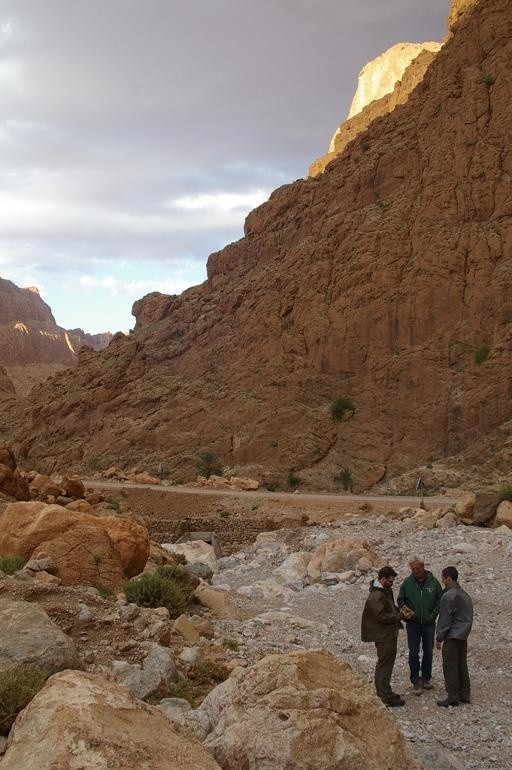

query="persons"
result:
[434,566,474,706]
[361,565,416,707]
[396,556,444,695]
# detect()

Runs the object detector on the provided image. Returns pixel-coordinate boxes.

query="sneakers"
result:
[410,681,434,696]
[376,689,405,706]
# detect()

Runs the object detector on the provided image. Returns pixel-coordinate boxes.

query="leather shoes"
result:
[436,697,459,707]
